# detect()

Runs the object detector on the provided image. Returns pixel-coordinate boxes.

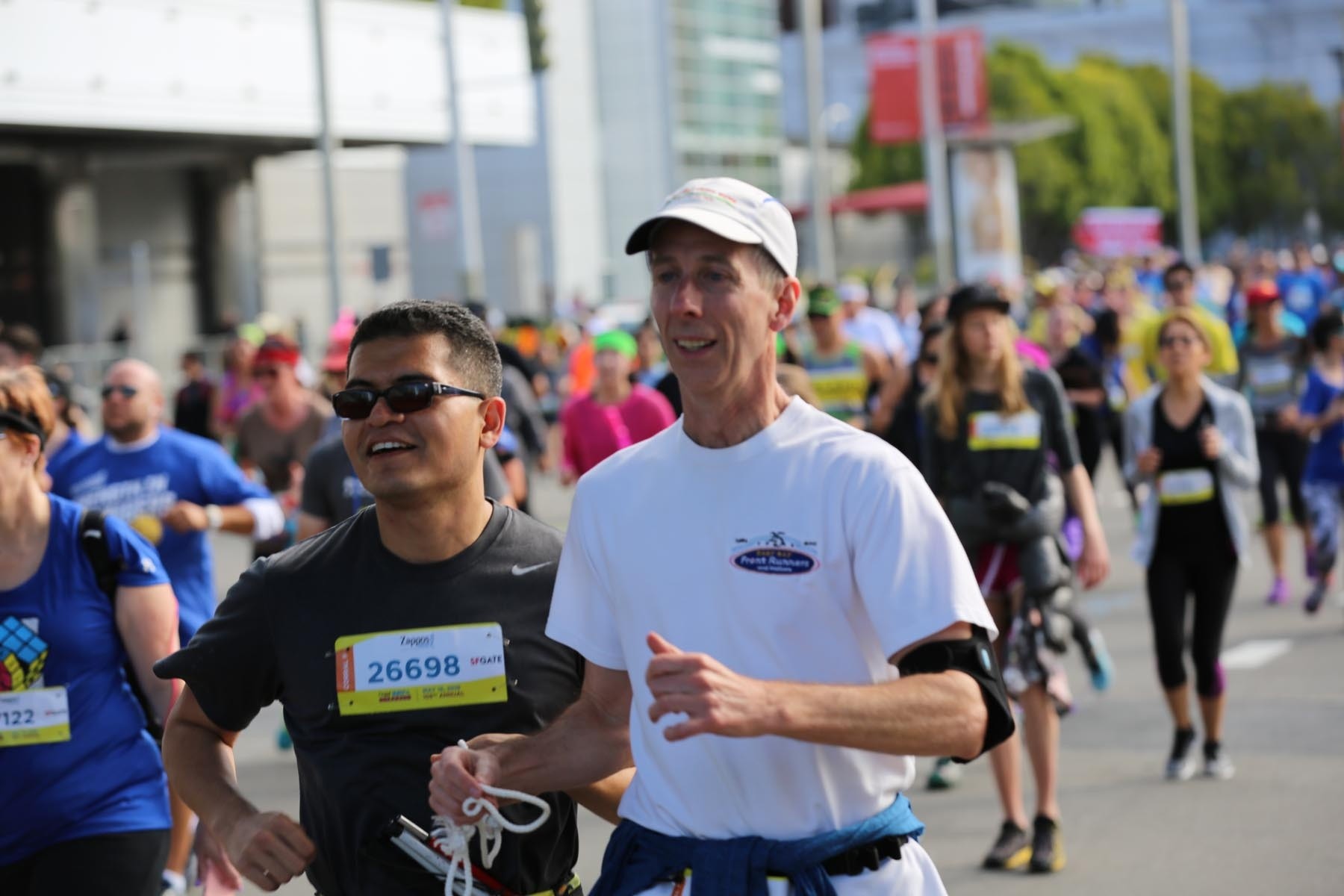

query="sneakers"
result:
[1029,816,1069,872]
[983,818,1033,869]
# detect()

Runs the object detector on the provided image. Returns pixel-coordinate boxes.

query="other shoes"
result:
[1304,584,1325,612]
[1200,739,1229,775]
[1165,725,1198,780]
[1070,624,1113,690]
[929,756,965,790]
[1268,579,1288,602]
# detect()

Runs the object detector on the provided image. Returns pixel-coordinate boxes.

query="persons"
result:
[428,179,1016,896]
[2,234,1343,875]
[0,340,180,896]
[152,306,639,896]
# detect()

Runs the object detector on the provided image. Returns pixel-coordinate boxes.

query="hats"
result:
[945,285,1010,321]
[624,176,798,280]
[805,287,842,315]
[1245,279,1278,305]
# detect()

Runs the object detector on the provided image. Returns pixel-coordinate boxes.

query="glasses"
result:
[332,378,485,421]
[1166,282,1192,292]
[100,384,157,400]
